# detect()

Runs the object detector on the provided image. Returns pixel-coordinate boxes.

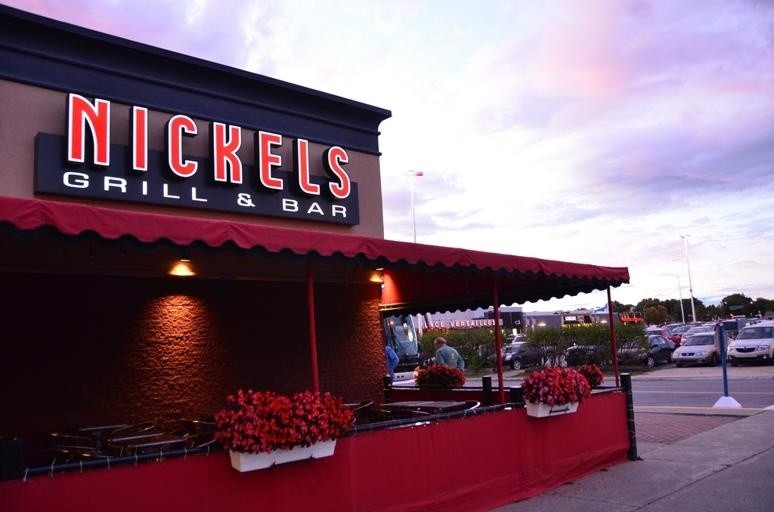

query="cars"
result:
[502,342,548,370]
[507,333,526,343]
[643,313,773,345]
[564,342,602,365]
[671,331,728,367]
[616,334,677,370]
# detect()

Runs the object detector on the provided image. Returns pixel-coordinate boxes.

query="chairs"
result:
[344,399,481,425]
[49,416,218,462]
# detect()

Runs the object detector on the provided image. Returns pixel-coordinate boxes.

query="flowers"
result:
[578,363,604,387]
[413,361,467,388]
[521,366,591,405]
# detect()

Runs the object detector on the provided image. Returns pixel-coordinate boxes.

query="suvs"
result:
[728,323,773,366]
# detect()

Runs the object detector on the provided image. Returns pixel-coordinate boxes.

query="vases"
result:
[525,400,578,419]
[228,435,337,473]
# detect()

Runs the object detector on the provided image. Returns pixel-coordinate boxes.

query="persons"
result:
[384,345,399,388]
[434,337,465,372]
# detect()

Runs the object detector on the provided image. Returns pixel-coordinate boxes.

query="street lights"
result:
[681,233,698,324]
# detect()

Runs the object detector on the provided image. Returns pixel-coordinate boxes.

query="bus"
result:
[383,311,423,390]
